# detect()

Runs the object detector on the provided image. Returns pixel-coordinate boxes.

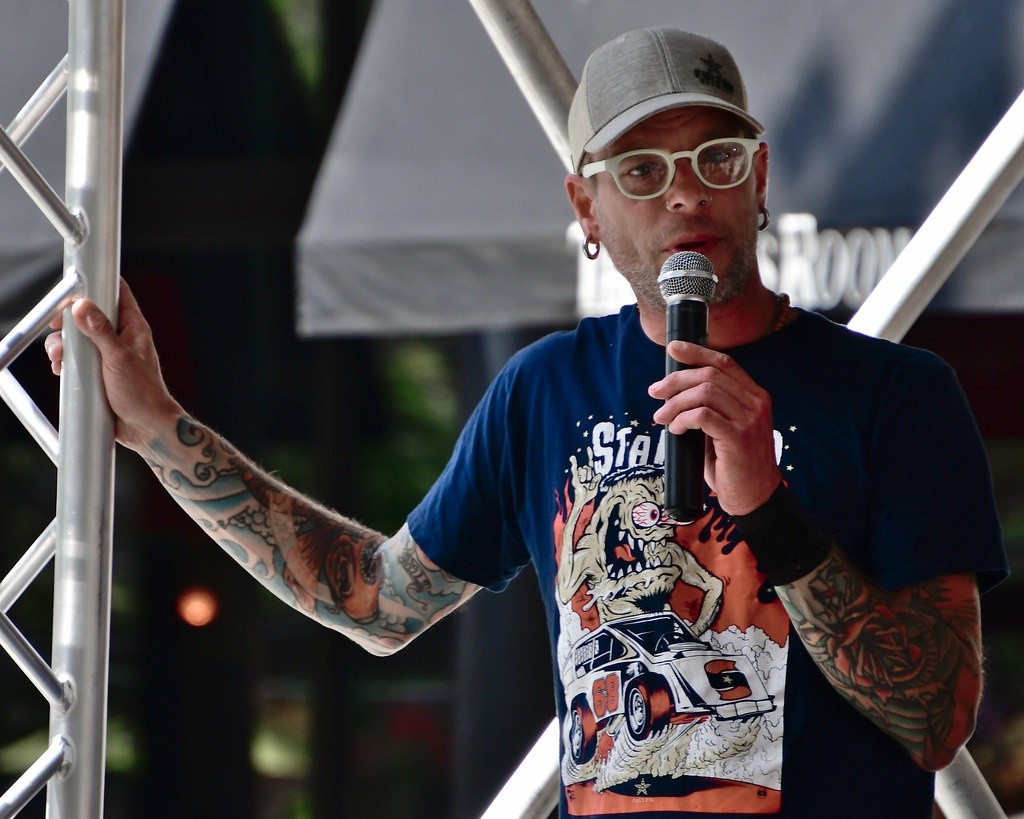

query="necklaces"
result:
[760,289,790,335]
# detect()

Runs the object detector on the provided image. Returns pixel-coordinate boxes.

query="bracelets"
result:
[728,480,833,587]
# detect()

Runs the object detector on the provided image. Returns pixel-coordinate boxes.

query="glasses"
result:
[581,137,761,200]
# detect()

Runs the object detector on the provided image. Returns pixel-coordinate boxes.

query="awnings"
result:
[0,0,182,333]
[291,1,1024,336]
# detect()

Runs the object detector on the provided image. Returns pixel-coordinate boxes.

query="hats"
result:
[567,27,767,174]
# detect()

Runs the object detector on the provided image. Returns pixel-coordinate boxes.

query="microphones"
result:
[659,250,718,522]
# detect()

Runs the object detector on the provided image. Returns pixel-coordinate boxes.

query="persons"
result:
[45,25,1009,819]
